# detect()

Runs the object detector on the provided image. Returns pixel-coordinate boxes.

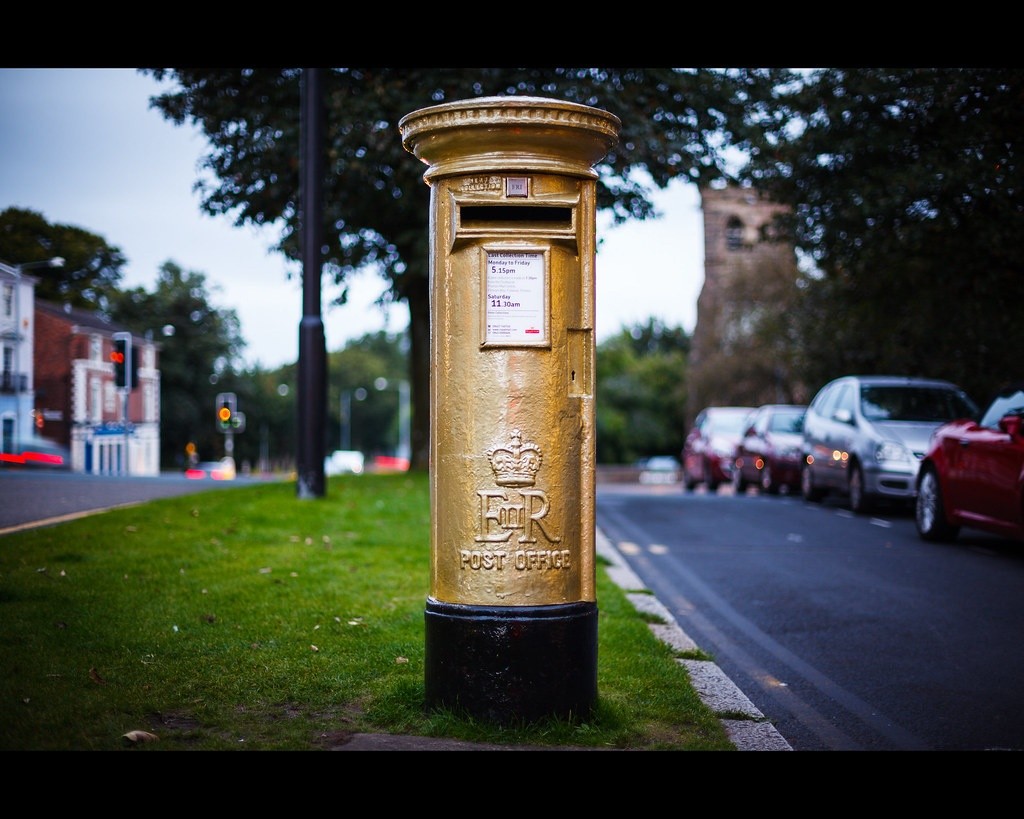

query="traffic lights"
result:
[215,392,237,431]
[111,331,130,389]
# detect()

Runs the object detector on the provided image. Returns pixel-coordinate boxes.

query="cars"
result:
[730,402,812,498]
[796,376,984,514]
[682,403,753,495]
[913,380,1024,544]
